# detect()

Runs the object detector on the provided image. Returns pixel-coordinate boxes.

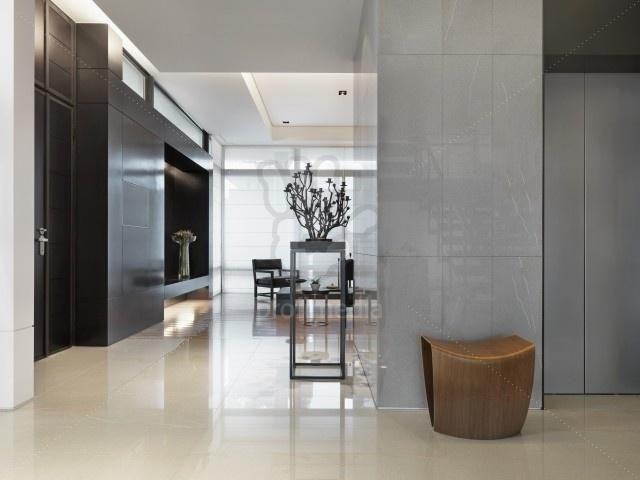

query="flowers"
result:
[171,230,197,275]
[284,162,350,239]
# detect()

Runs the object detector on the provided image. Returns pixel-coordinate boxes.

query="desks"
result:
[276,287,353,315]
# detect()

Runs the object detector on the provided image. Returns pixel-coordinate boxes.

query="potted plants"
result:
[310,278,320,291]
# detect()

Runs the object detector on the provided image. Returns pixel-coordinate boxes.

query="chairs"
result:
[252,259,299,301]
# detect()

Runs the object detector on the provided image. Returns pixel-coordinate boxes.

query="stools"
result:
[421,335,535,440]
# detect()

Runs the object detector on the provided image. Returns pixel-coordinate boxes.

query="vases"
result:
[178,242,190,281]
